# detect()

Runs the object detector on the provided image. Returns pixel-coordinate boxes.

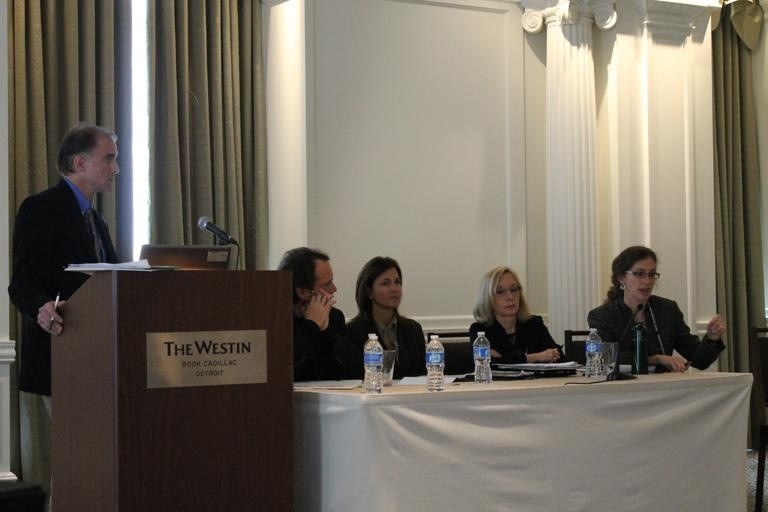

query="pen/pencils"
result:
[556,343,565,351]
[685,360,692,366]
[49,290,62,330]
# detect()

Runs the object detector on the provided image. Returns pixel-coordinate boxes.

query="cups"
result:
[382,350,396,387]
[602,342,619,378]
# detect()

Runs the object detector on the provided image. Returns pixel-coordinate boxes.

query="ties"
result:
[85,208,107,266]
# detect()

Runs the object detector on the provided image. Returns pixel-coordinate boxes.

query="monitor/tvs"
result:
[140,245,237,269]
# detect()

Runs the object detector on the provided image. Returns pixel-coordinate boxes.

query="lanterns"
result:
[587,246,727,374]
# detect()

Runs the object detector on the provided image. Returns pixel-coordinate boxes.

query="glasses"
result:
[622,270,659,279]
[490,286,521,296]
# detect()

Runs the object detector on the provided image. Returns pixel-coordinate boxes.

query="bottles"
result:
[585,328,602,380]
[426,335,444,392]
[473,332,492,385]
[364,333,384,394]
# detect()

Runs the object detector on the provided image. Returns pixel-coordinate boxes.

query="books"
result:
[491,361,581,379]
[64,259,176,272]
[293,380,363,389]
[399,374,475,384]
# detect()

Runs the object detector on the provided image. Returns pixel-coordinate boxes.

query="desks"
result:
[291,369,756,512]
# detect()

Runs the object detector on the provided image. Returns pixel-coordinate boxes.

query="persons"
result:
[278,248,345,382]
[337,257,426,380]
[8,124,120,512]
[469,266,568,373]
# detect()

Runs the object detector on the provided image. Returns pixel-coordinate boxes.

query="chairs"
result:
[564,328,595,368]
[746,323,768,511]
[425,330,481,377]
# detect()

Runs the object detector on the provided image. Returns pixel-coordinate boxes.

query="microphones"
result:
[606,302,644,381]
[197,215,237,244]
